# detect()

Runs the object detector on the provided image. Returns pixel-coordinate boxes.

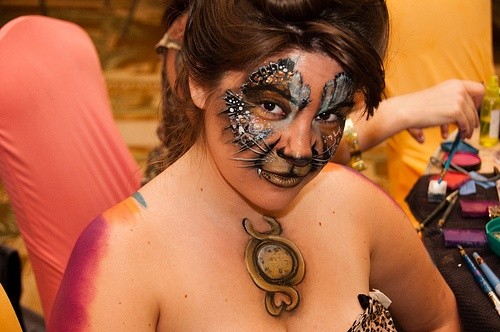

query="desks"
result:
[398,128,500,332]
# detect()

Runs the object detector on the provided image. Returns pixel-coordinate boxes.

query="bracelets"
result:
[344,114,366,172]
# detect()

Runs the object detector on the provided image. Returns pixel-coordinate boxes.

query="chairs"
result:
[0,14,142,332]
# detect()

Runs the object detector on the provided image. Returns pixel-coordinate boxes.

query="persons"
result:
[141,0,487,189]
[48,0,461,332]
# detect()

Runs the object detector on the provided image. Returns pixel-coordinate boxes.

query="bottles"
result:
[478,75,500,148]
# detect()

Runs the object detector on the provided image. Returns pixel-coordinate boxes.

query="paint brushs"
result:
[457,243,500,315]
[417,128,500,231]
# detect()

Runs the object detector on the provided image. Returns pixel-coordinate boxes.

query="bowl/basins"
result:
[485,217,500,256]
